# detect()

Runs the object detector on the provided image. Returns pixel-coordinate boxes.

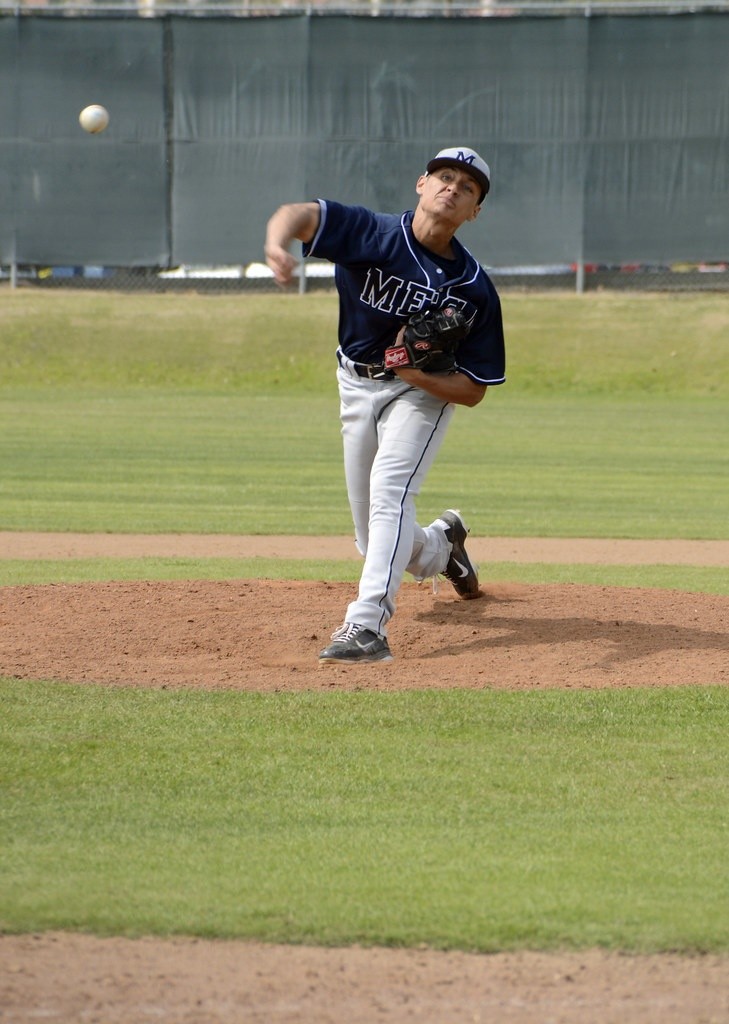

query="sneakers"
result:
[432,509,481,600]
[319,622,393,668]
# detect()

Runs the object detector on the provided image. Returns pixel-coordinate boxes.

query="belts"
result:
[336,350,395,381]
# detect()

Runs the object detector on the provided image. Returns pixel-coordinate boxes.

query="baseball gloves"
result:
[381,307,464,374]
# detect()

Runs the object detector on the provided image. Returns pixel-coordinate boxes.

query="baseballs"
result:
[80,104,111,134]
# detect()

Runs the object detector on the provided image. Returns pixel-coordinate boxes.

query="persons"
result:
[265,147,506,664]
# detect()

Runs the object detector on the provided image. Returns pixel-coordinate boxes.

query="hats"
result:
[425,147,490,206]
